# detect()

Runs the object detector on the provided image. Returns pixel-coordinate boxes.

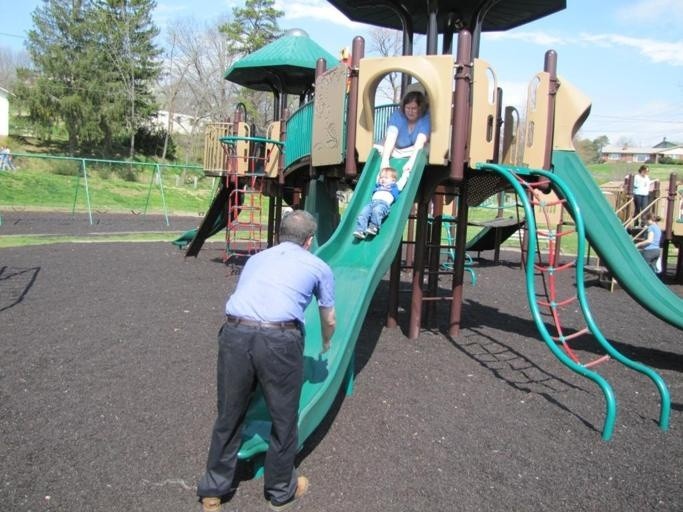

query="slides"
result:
[173,175,245,246]
[549,151,682,329]
[236,149,428,463]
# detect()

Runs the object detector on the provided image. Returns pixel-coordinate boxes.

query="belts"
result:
[226,316,296,330]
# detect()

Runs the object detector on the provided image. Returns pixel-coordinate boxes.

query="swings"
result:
[10,156,212,216]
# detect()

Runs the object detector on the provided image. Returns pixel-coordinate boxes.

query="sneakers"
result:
[200,495,222,512]
[268,476,309,510]
[353,226,377,239]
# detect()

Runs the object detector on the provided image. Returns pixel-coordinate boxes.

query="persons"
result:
[632,165,658,228]
[373,91,430,171]
[0,145,16,172]
[192,206,338,510]
[352,155,410,242]
[634,214,663,274]
